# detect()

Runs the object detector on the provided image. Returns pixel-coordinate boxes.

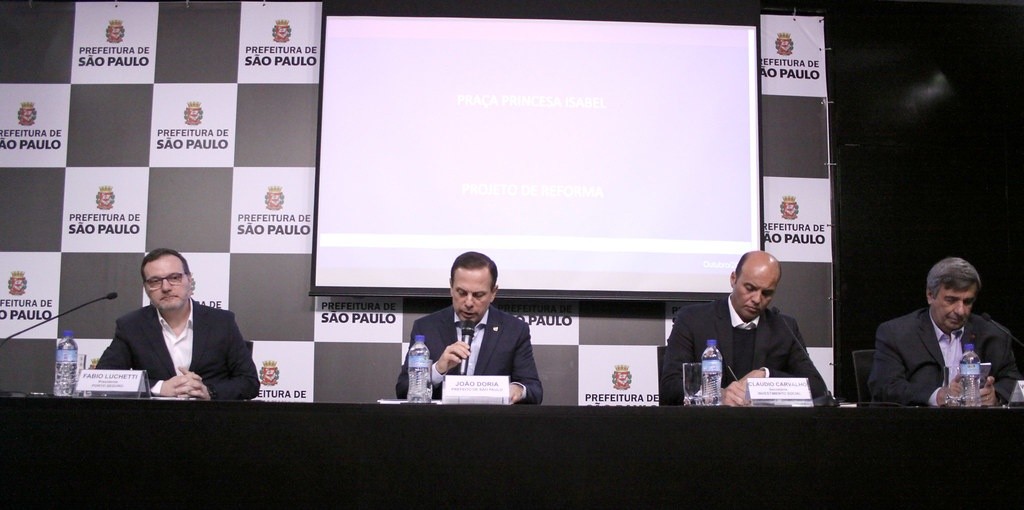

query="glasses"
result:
[143,273,185,291]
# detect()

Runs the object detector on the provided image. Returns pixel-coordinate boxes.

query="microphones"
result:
[772,306,841,407]
[459,319,476,375]
[982,313,1024,348]
[0,292,119,350]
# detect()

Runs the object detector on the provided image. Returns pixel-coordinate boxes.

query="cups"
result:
[72,354,86,398]
[944,366,965,408]
[682,363,705,405]
[416,359,433,404]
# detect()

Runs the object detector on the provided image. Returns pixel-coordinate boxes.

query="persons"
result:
[95,248,260,401]
[660,250,826,407]
[871,257,1023,406]
[396,252,544,404]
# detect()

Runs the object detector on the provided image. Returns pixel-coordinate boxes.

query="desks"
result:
[0,397,1024,510]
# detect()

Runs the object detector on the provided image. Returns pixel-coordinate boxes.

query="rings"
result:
[986,396,989,401]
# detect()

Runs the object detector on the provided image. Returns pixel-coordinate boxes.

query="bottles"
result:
[959,344,981,407]
[53,330,78,396]
[701,339,722,406]
[408,335,430,402]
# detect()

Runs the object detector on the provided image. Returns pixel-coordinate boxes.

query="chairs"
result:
[853,348,904,407]
